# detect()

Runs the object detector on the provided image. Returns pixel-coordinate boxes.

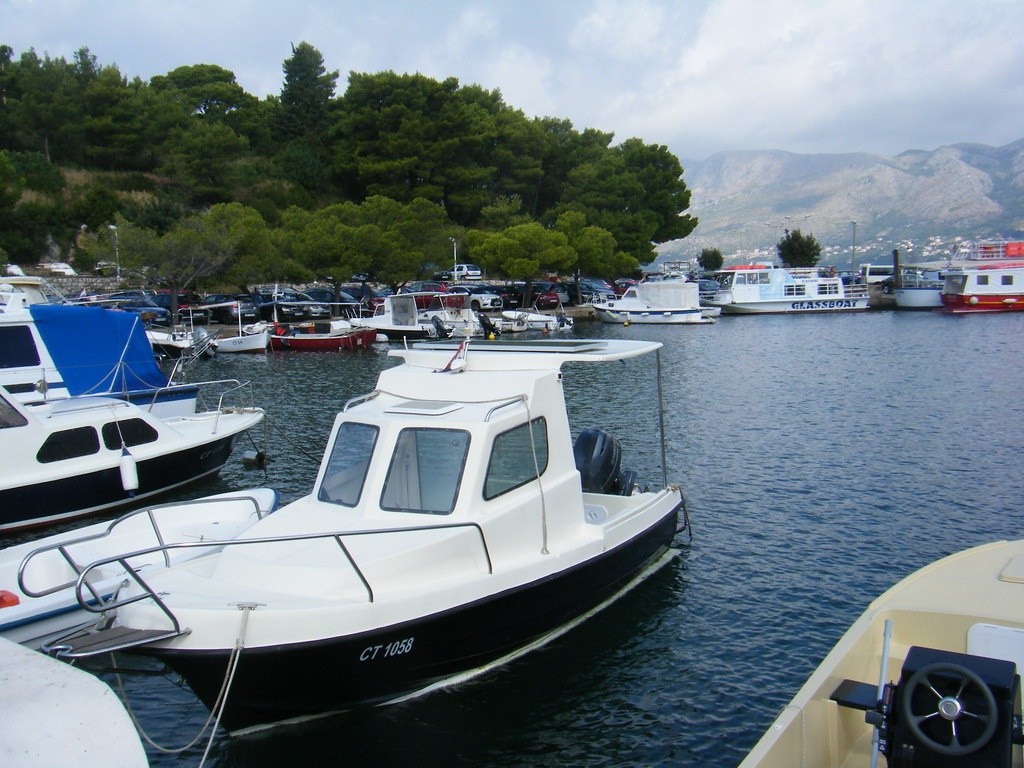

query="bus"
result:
[861,266,894,285]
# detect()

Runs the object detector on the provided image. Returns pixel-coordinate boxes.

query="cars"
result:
[110,295,171,329]
[204,292,262,325]
[484,282,617,309]
[447,285,504,311]
[835,271,862,287]
[0,487,278,652]
[150,290,212,325]
[251,282,463,323]
[420,263,452,281]
[881,274,923,294]
[611,274,721,299]
[448,264,483,281]
[350,273,370,282]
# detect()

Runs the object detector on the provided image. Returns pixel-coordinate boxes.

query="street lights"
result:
[109,225,120,281]
[851,220,856,284]
[450,237,457,281]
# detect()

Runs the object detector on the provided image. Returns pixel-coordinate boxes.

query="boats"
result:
[0,379,265,536]
[502,293,574,331]
[736,538,1024,768]
[238,301,377,351]
[0,276,200,417]
[940,241,1024,314]
[18,336,684,741]
[349,291,476,339]
[418,292,533,332]
[893,266,944,311]
[145,300,269,355]
[700,268,870,314]
[591,282,715,324]
